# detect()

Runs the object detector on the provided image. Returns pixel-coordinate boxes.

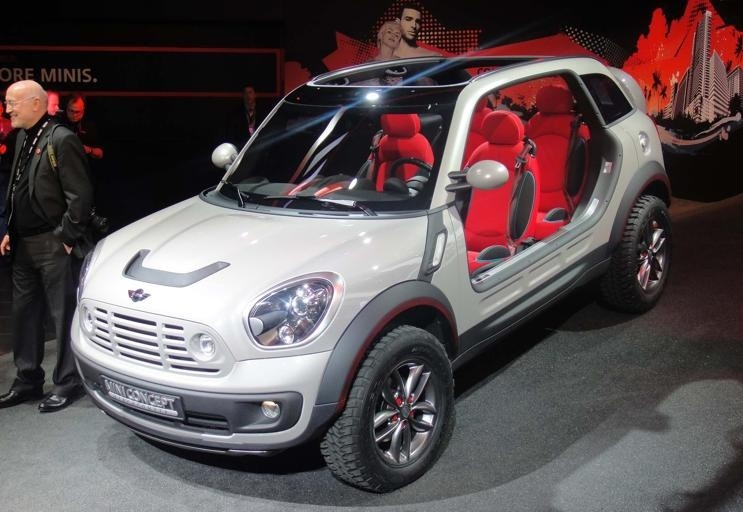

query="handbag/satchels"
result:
[57,207,110,259]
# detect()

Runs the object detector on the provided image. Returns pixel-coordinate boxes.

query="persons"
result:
[57,91,104,172]
[359,21,407,89]
[0,100,17,145]
[222,81,266,150]
[0,80,94,412]
[394,2,441,58]
[44,89,59,118]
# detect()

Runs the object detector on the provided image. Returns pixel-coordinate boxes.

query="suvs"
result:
[69,53,674,489]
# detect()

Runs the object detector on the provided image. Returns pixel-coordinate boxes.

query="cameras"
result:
[87,207,109,234]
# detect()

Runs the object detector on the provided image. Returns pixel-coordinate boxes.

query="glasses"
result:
[4,96,34,107]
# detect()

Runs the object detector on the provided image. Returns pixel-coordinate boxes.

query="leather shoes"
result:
[39,389,81,412]
[0,390,43,408]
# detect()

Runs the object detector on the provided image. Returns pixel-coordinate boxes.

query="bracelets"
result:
[89,146,93,155]
